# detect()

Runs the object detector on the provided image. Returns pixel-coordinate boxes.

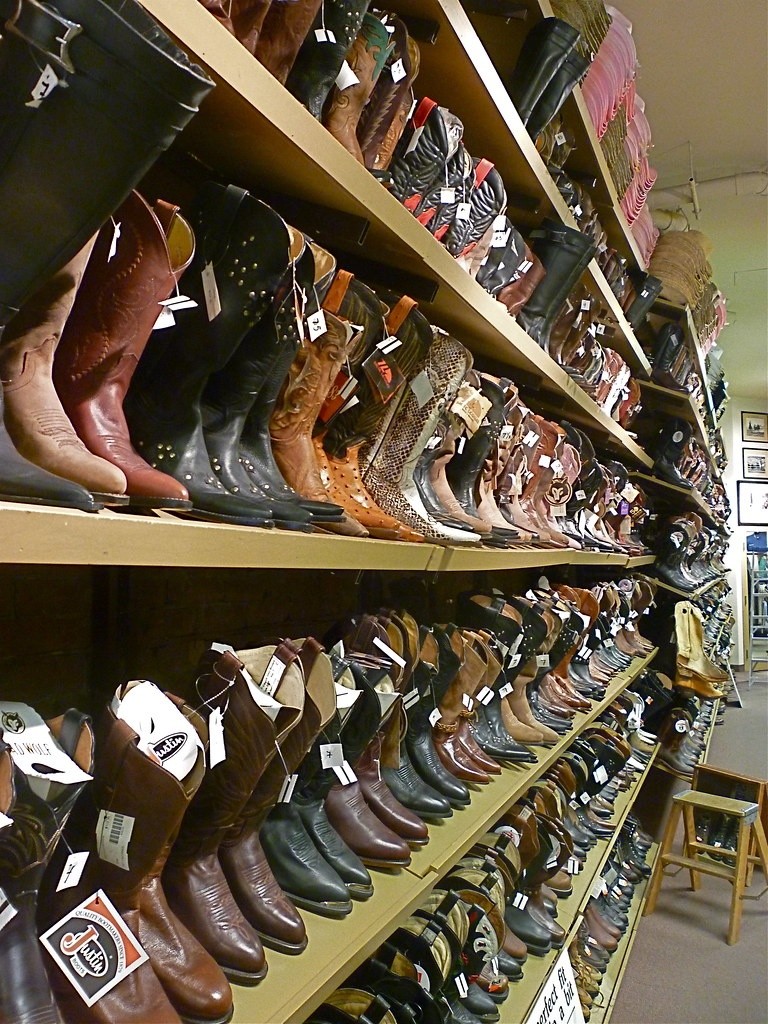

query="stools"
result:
[640,784,768,946]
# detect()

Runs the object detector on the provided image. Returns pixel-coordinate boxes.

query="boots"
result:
[0,0,661,1023]
[629,321,735,778]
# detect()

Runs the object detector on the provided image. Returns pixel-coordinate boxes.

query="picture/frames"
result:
[4,1,746,1023]
[736,479,768,525]
[740,447,768,481]
[740,410,768,444]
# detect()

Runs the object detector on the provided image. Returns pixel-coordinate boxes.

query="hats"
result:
[548,0,730,427]
